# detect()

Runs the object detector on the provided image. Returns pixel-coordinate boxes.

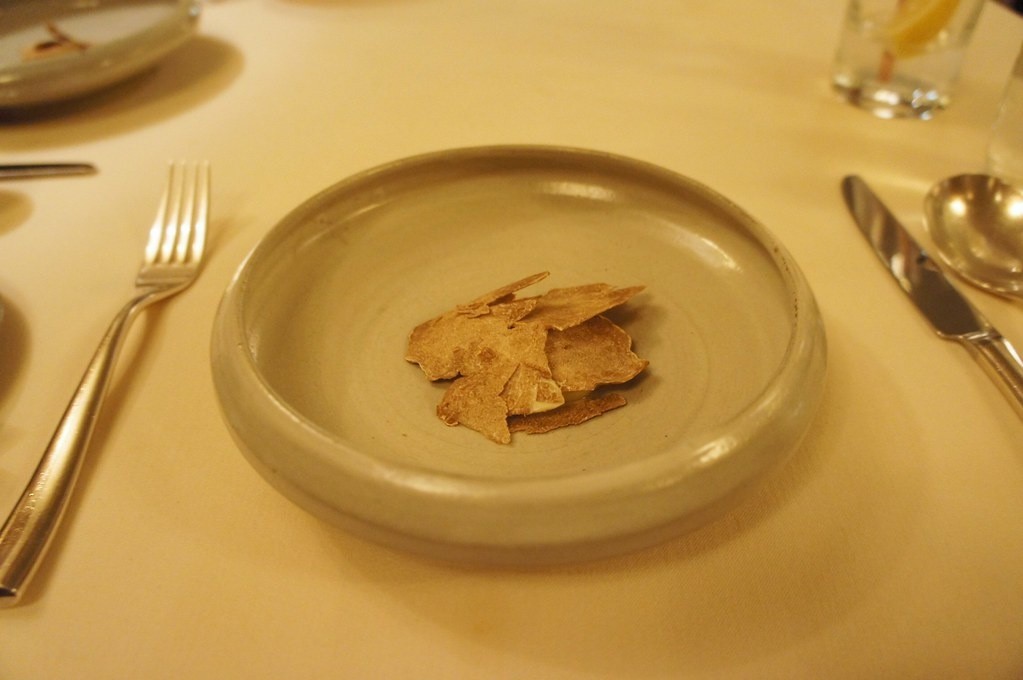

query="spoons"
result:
[921,175,1022,310]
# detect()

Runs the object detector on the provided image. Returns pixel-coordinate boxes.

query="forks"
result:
[1,156,211,603]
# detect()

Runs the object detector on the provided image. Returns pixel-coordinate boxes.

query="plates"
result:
[208,142,826,557]
[0,2,204,117]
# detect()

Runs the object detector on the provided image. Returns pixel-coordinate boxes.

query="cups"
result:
[822,0,987,123]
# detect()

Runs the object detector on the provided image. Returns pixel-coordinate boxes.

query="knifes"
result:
[840,172,1021,425]
